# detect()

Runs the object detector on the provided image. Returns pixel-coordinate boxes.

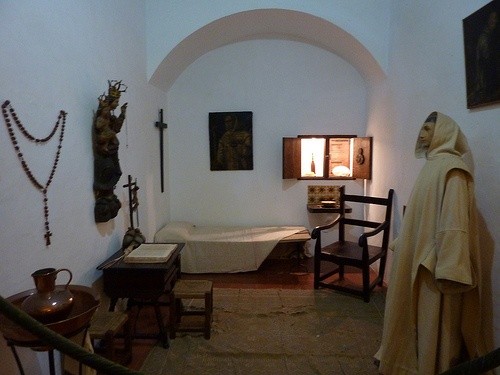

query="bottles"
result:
[311,153,316,175]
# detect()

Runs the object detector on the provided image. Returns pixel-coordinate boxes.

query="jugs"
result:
[21,268,74,323]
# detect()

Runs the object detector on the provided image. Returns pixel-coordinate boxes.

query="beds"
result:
[155,226,311,274]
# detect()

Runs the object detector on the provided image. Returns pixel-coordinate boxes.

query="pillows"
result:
[156,222,196,241]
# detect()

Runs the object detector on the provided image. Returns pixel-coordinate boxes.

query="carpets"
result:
[140,289,386,375]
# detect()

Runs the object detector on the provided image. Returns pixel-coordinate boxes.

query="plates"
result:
[332,166,350,176]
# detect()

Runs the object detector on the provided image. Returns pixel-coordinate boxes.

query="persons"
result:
[95,82,128,200]
[374,112,493,375]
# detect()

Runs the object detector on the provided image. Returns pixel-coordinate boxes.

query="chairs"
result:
[312,186,395,302]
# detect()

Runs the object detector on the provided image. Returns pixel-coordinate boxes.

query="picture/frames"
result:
[209,111,253,170]
[462,0,500,109]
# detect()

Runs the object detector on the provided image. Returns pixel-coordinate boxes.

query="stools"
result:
[89,310,127,363]
[169,280,213,339]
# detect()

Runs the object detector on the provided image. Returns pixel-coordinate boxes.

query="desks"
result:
[0,323,101,375]
[94,241,185,348]
